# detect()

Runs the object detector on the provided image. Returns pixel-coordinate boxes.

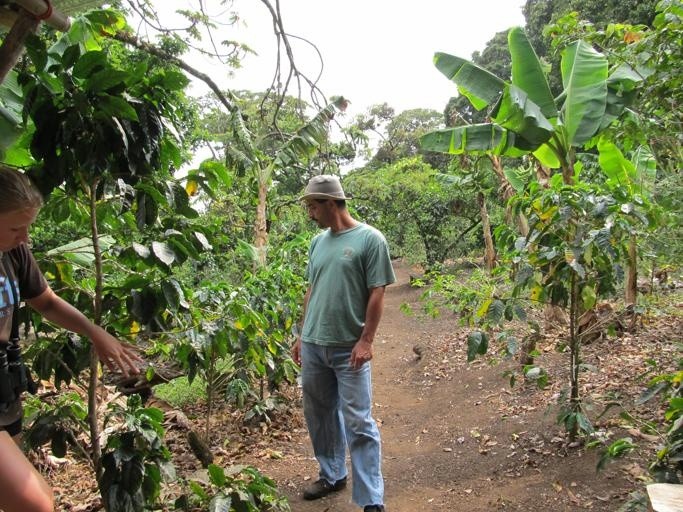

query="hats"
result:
[297,174,352,201]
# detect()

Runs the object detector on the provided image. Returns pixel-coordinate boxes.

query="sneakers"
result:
[364,504,385,512]
[304,476,346,500]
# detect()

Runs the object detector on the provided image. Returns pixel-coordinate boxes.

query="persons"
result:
[0,163,148,511]
[288,173,397,512]
